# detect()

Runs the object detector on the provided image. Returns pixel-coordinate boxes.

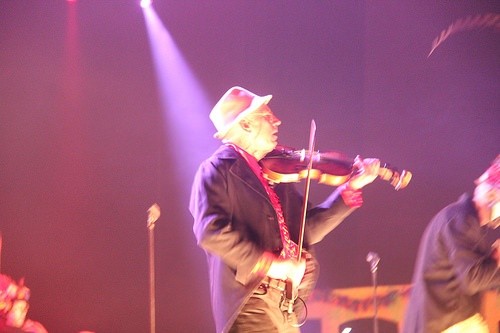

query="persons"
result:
[400,153,500,333]
[0,274,47,333]
[189,85,380,333]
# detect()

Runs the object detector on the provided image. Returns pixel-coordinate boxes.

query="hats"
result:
[209,86,272,139]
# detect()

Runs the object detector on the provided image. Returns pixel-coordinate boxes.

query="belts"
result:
[258,276,287,292]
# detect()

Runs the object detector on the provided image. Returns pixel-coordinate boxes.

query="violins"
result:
[260,143,412,192]
